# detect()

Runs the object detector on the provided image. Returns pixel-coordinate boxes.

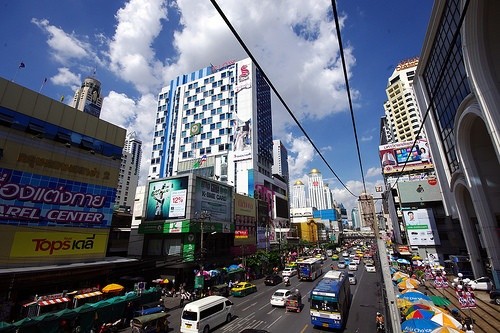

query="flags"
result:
[199,155,206,165]
[193,160,199,168]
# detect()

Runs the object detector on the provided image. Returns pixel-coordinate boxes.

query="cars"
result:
[316,252,326,261]
[348,273,357,284]
[470,277,493,291]
[270,289,290,307]
[231,281,256,297]
[264,274,282,286]
[282,262,298,277]
[332,243,377,273]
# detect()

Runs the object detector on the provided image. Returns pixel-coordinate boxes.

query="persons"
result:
[407,212,418,225]
[156,273,241,307]
[376,313,384,330]
[420,148,428,159]
[153,191,165,218]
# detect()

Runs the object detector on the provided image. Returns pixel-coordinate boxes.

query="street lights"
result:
[451,272,478,333]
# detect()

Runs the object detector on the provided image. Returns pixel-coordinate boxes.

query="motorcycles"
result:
[284,280,290,286]
[131,313,171,333]
[376,321,385,333]
[285,296,301,312]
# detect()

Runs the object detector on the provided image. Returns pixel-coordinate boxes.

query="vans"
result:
[327,250,332,256]
[180,295,235,333]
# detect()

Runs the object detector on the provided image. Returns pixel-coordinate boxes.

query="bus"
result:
[308,270,350,329]
[299,259,322,281]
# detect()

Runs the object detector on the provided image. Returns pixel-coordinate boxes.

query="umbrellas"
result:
[195,263,250,280]
[102,284,124,293]
[388,254,474,333]
[153,275,175,284]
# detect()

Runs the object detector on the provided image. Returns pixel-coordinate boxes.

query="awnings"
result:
[74,290,102,299]
[37,297,71,305]
